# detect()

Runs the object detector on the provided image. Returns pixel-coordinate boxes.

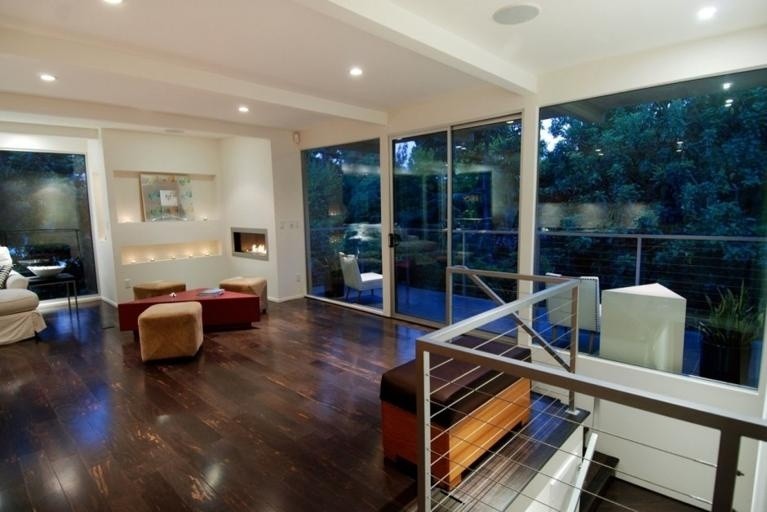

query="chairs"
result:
[338,250,382,303]
[543,271,603,353]
[0,246,46,346]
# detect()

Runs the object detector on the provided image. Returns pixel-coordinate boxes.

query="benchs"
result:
[379,332,535,491]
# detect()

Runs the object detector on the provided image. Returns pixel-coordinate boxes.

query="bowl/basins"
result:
[16,258,50,265]
[27,266,66,276]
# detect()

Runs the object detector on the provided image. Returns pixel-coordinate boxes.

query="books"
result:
[199,287,224,297]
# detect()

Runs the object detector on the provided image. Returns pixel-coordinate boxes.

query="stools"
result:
[136,301,204,363]
[219,275,267,316]
[133,282,185,298]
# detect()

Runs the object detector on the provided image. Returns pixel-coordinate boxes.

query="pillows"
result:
[0,262,18,290]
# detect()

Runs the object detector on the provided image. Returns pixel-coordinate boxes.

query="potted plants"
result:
[314,241,346,299]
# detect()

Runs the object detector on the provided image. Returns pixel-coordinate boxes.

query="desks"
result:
[114,286,259,334]
[25,273,78,313]
